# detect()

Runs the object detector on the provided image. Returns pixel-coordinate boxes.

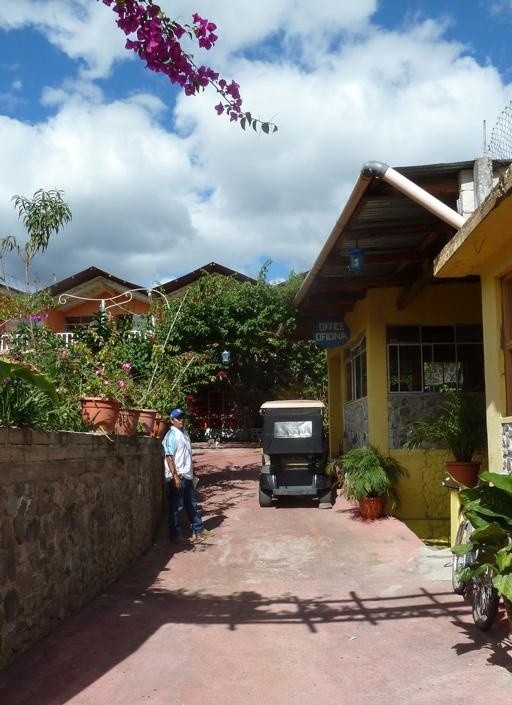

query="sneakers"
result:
[169,536,191,546]
[192,528,215,540]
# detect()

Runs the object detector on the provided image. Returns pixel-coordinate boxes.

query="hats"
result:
[170,408,186,420]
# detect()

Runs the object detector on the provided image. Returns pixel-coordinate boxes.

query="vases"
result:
[81,395,120,434]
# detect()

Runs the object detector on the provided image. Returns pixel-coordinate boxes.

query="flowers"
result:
[81,360,131,398]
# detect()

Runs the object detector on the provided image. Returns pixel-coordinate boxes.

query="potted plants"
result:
[404,375,482,488]
[116,325,191,439]
[341,443,407,518]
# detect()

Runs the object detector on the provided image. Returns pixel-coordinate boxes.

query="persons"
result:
[161,409,215,544]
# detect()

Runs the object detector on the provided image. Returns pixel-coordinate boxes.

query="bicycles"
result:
[441,476,501,630]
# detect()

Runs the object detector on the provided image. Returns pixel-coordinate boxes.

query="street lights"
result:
[220,350,230,430]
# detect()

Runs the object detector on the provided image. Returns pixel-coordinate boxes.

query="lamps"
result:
[347,248,367,272]
